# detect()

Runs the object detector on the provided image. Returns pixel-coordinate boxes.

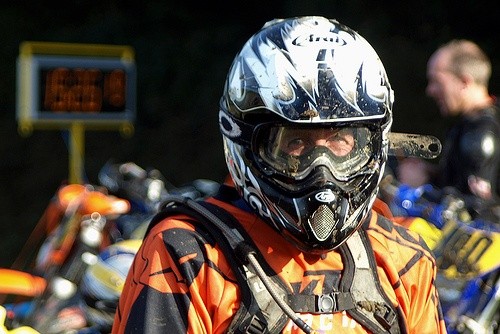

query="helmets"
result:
[218,16,394,255]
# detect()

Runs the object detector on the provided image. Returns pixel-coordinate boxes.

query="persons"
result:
[114,16,451,334]
[0,41,500,334]
